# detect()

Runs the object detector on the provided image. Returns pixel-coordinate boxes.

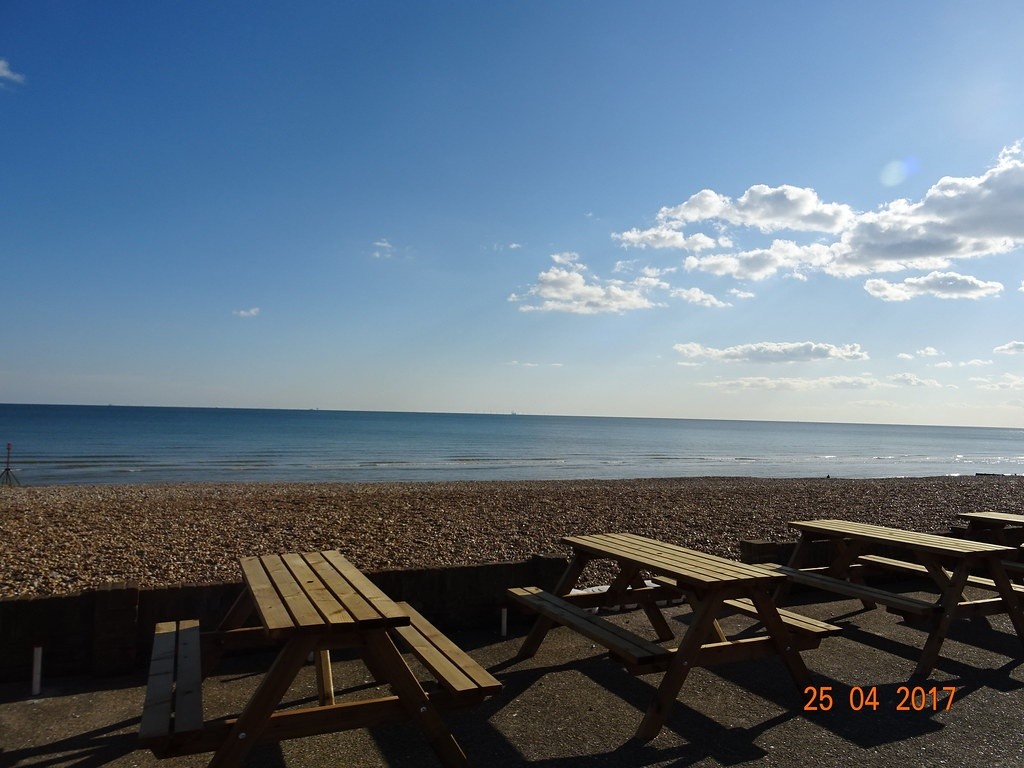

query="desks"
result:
[771,518,1024,688]
[151,549,503,768]
[942,512,1024,586]
[519,534,824,742]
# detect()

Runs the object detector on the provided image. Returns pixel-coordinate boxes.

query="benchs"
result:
[857,553,1024,597]
[754,561,939,615]
[653,576,843,638]
[508,585,673,666]
[140,619,203,736]
[392,601,503,698]
